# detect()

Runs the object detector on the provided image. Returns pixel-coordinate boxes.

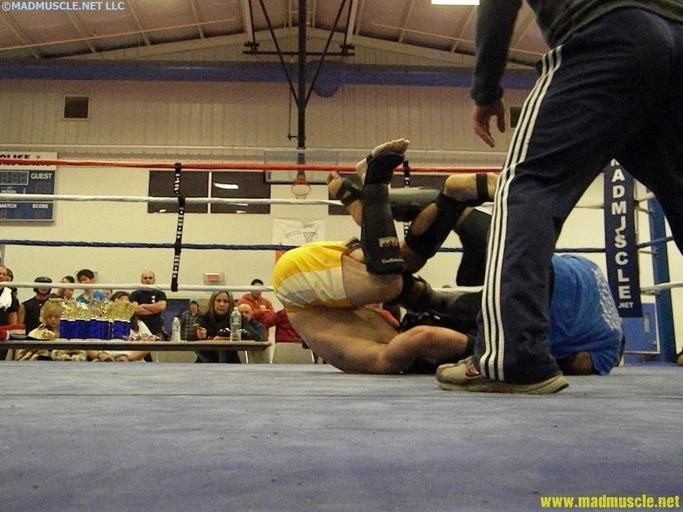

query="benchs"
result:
[149,338,315,365]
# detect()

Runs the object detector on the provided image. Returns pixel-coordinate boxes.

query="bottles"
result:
[229,307,242,341]
[171,317,181,341]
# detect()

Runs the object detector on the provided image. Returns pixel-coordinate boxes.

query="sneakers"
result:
[434,354,569,394]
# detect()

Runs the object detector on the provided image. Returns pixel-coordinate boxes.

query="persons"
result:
[264,138,499,379]
[0,261,318,362]
[436,1,681,397]
[320,165,627,377]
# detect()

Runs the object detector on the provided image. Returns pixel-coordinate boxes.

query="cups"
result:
[57,317,130,340]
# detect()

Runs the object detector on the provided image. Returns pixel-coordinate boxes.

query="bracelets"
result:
[466,334,480,360]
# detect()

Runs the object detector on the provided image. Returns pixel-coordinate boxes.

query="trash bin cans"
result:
[622,302,659,365]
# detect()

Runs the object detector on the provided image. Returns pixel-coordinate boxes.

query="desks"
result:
[0,337,271,362]
